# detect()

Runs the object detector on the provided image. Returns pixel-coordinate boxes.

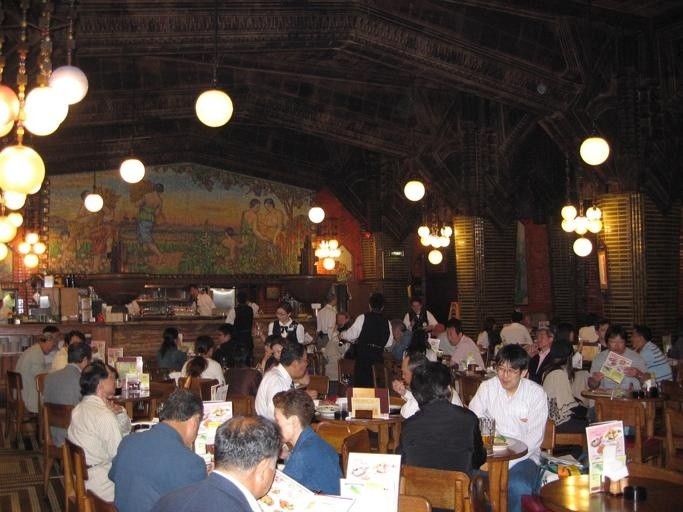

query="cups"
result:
[479,418,497,455]
[114,379,122,395]
[40,314,47,322]
[334,401,348,421]
[174,306,195,316]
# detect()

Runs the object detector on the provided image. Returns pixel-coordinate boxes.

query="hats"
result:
[551,338,571,357]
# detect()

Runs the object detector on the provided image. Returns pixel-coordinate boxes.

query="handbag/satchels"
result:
[541,455,584,488]
[570,396,587,417]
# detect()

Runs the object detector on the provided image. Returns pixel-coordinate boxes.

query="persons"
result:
[113,303,126,322]
[262,198,284,273]
[241,199,269,272]
[316,292,484,421]
[476,312,674,433]
[12,335,57,414]
[396,362,487,474]
[102,366,131,436]
[40,343,92,459]
[134,182,163,255]
[68,364,123,502]
[43,325,65,367]
[223,228,247,272]
[128,296,140,318]
[468,344,549,511]
[51,331,86,373]
[253,302,341,495]
[108,389,208,510]
[156,284,260,385]
[150,413,281,511]
[79,190,92,216]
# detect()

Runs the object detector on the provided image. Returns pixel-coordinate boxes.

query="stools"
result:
[448,301,461,320]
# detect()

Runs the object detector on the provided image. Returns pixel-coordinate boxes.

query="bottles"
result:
[628,383,634,399]
[65,273,75,287]
[607,473,630,497]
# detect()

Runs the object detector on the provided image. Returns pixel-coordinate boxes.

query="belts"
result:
[366,343,382,350]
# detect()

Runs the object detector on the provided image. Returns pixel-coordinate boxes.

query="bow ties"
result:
[280,326,288,333]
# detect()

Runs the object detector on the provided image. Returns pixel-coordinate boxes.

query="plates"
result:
[316,405,339,416]
[493,439,516,450]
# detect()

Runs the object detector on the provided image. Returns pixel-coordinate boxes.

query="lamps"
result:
[404,181,425,202]
[580,120,610,166]
[418,201,453,266]
[0,0,89,262]
[120,158,146,184]
[193,85,234,128]
[84,192,104,212]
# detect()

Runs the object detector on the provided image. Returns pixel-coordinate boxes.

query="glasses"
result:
[497,365,520,376]
[633,390,644,399]
